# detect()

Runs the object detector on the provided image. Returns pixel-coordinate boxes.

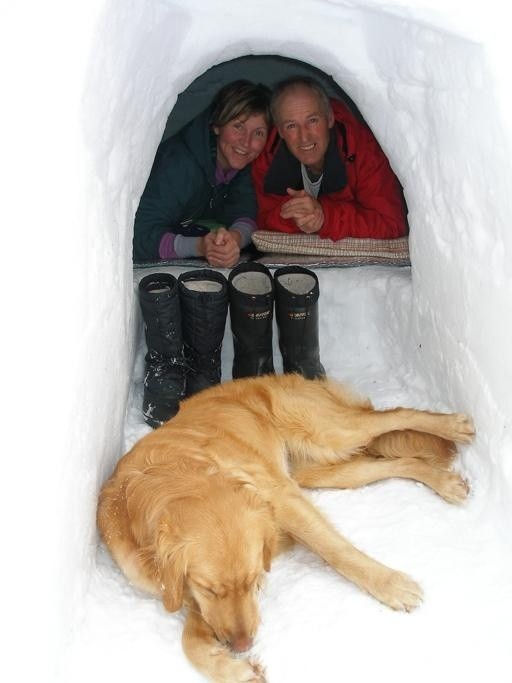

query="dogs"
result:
[97,371,476,683]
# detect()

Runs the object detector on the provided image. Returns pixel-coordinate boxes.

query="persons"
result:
[251,74,409,240]
[131,76,274,269]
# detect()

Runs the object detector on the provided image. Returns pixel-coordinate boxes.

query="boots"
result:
[139,273,183,428]
[178,269,228,400]
[274,265,326,380]
[229,262,275,379]
